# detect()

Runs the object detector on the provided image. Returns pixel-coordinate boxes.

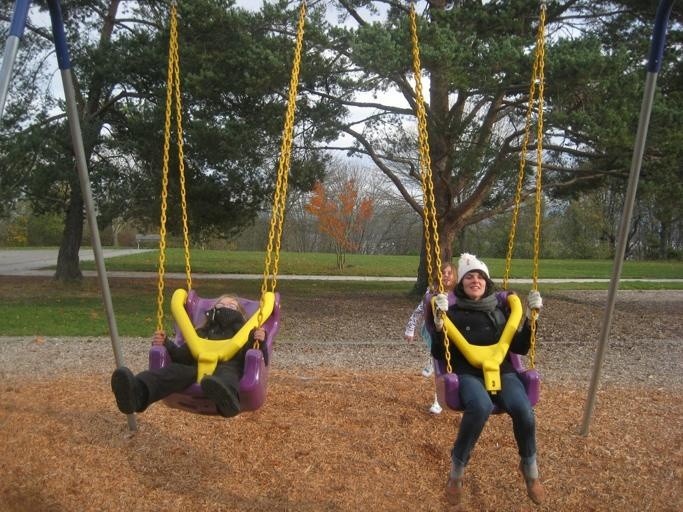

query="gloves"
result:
[527,290,544,309]
[434,292,449,311]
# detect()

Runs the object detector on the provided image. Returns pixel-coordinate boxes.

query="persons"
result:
[110,292,268,417]
[404,262,458,416]
[430,252,546,504]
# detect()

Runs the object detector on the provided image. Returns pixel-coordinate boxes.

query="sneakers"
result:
[445,477,463,506]
[429,403,443,414]
[422,367,434,378]
[201,376,240,417]
[519,461,544,504]
[111,368,136,414]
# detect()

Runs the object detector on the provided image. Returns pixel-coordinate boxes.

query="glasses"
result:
[214,303,238,310]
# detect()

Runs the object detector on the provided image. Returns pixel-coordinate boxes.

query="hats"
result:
[458,252,490,284]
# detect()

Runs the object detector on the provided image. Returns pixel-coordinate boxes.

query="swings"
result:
[150,1,309,415]
[410,0,547,413]
